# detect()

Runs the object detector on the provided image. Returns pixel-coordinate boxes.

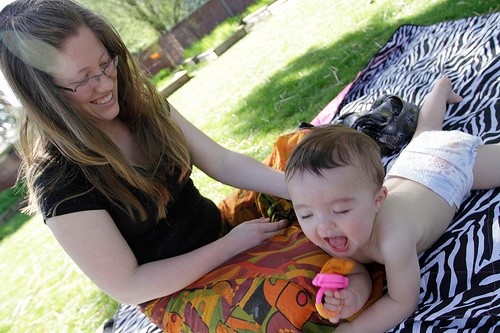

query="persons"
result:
[285,77,500,333]
[0,0,334,333]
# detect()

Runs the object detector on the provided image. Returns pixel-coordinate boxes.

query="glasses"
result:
[54,54,118,95]
